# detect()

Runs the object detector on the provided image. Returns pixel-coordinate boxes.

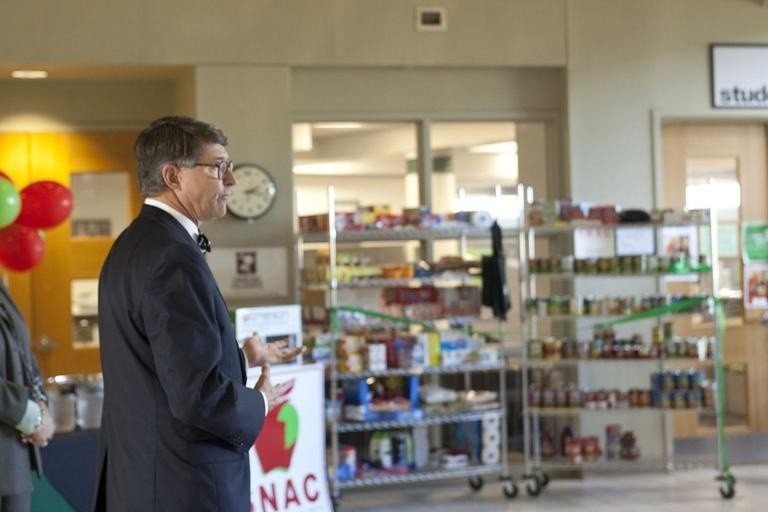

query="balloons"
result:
[0,171,73,273]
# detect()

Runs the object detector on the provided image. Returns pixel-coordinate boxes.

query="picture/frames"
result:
[709,43,768,111]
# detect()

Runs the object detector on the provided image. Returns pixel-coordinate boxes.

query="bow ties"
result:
[198,231,212,253]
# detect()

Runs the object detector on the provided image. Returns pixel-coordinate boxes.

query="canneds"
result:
[527,256,714,408]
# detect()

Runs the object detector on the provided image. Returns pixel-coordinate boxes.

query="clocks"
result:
[226,163,277,220]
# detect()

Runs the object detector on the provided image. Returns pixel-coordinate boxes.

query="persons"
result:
[99,116,307,512]
[0,280,56,511]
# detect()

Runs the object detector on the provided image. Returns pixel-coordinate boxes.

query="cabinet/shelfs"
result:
[294,186,516,496]
[524,186,736,500]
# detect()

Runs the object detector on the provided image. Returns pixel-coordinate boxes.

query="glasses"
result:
[173,161,234,179]
